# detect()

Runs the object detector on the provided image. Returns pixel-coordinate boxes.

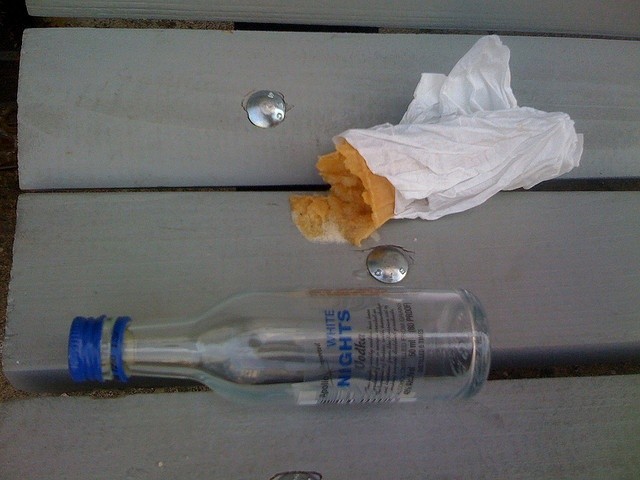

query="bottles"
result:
[67,286,493,409]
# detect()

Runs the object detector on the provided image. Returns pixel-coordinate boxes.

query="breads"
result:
[290,34,585,250]
